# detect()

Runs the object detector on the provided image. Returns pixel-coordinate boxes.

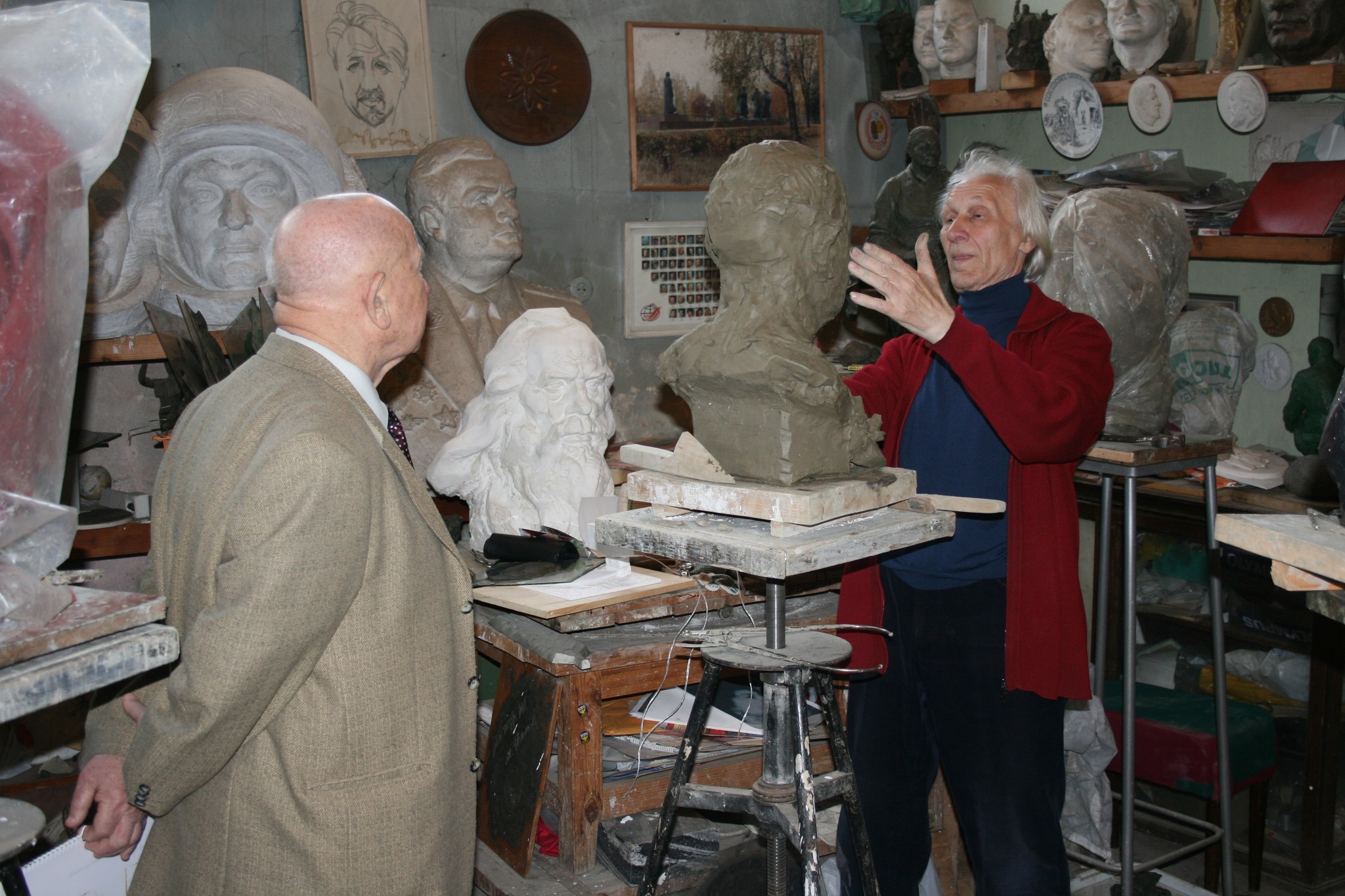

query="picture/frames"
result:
[626,21,825,191]
[624,220,721,338]
[302,0,438,158]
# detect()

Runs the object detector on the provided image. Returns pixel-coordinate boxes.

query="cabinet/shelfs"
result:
[851,61,1345,263]
[69,331,228,560]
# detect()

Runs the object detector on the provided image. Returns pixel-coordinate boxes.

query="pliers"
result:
[1306,507,1345,530]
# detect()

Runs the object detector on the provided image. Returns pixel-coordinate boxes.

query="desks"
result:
[473,592,850,875]
[1065,432,1235,896]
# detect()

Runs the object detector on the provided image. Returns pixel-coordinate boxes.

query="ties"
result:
[387,406,414,468]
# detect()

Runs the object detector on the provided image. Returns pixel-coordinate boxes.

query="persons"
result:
[83,67,370,341]
[932,0,978,80]
[867,126,950,268]
[430,309,616,553]
[63,191,478,896]
[911,4,943,87]
[1283,336,1344,456]
[1106,0,1180,75]
[1013,0,1037,64]
[840,143,1117,896]
[655,139,887,484]
[876,10,924,92]
[1042,1,1112,81]
[378,138,592,478]
[1237,0,1345,65]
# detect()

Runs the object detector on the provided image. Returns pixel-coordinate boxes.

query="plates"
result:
[856,100,893,161]
[1041,72,1103,160]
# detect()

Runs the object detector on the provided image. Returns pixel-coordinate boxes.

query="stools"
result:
[1102,677,1279,893]
